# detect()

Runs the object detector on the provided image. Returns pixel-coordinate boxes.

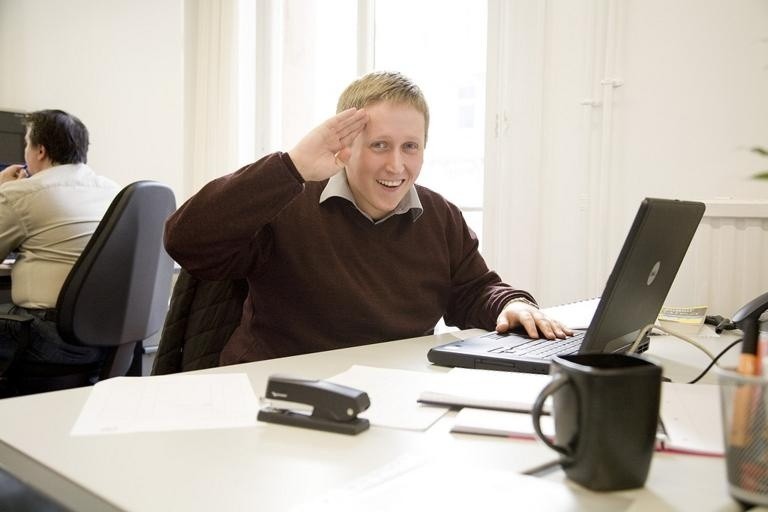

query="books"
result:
[656,305,709,337]
[448,366,726,458]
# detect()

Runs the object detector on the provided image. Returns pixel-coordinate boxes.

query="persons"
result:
[0,107,128,367]
[160,69,575,368]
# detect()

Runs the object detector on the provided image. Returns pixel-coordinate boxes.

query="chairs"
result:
[0,179,179,400]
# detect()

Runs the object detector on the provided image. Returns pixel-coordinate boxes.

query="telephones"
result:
[730,291,768,330]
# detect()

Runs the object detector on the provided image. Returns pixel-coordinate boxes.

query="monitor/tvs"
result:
[426,198,706,378]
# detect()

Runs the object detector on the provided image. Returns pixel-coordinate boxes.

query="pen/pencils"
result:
[24,165,27,169]
[726,320,759,485]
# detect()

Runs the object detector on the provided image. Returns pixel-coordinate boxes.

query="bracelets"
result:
[500,297,539,312]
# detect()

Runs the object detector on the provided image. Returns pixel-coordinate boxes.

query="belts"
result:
[24,308,57,321]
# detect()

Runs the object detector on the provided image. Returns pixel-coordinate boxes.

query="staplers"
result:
[257,378,371,435]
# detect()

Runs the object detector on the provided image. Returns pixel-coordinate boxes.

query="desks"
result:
[0,300,768,511]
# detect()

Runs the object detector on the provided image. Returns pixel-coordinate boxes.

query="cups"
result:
[533,350,662,494]
